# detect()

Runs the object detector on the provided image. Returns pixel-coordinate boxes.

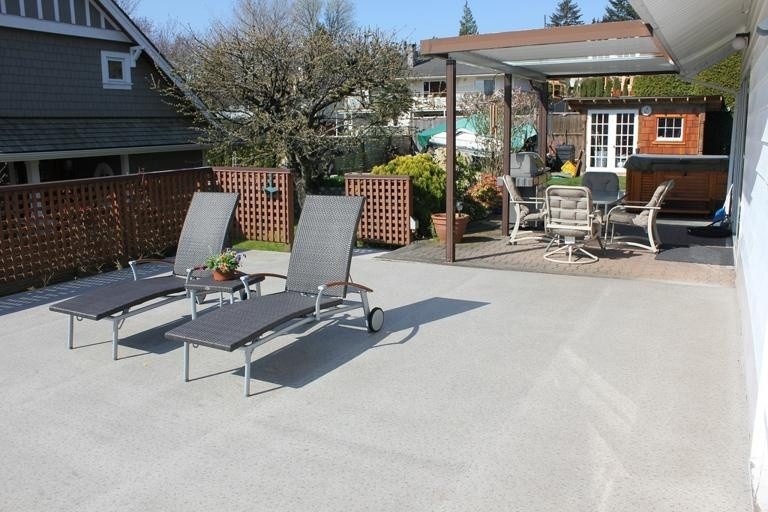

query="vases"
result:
[210,270,233,281]
[431,213,471,244]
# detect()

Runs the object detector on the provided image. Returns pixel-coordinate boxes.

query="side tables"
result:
[184,274,265,321]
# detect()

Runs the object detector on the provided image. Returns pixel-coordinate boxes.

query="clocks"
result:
[640,104,653,116]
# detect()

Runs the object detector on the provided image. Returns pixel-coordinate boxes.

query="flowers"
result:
[206,248,241,274]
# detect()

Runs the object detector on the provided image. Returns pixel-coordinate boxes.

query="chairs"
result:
[49,193,240,360]
[503,171,675,265]
[164,194,384,398]
